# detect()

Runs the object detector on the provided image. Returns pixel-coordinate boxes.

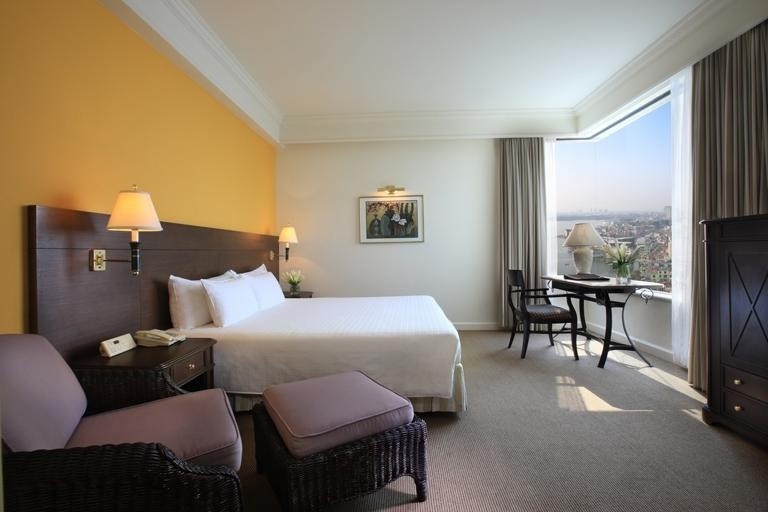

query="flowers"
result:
[279,270,305,286]
[602,238,641,278]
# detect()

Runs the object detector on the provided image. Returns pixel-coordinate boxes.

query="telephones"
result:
[135,329,187,346]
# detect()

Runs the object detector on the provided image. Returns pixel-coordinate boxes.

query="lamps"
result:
[562,223,609,281]
[89,185,163,276]
[268,224,297,262]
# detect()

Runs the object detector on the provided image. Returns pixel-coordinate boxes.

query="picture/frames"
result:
[358,195,424,243]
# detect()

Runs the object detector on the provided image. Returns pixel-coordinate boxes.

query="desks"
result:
[541,274,666,368]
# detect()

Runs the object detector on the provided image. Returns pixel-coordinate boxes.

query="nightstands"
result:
[64,334,217,417]
[282,291,313,298]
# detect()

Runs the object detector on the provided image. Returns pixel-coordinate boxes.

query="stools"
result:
[252,370,427,512]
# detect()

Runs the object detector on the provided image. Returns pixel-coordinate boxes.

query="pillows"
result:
[0,333,87,452]
[200,276,259,328]
[167,269,238,332]
[247,271,286,310]
[237,264,268,280]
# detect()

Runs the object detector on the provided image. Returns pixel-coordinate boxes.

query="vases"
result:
[616,263,630,286]
[290,285,300,297]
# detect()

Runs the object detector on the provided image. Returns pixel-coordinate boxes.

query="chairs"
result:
[0,333,242,511]
[504,269,580,361]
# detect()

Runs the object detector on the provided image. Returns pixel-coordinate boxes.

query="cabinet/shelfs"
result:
[698,213,768,450]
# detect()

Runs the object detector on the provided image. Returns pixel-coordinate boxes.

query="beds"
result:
[24,205,469,415]
